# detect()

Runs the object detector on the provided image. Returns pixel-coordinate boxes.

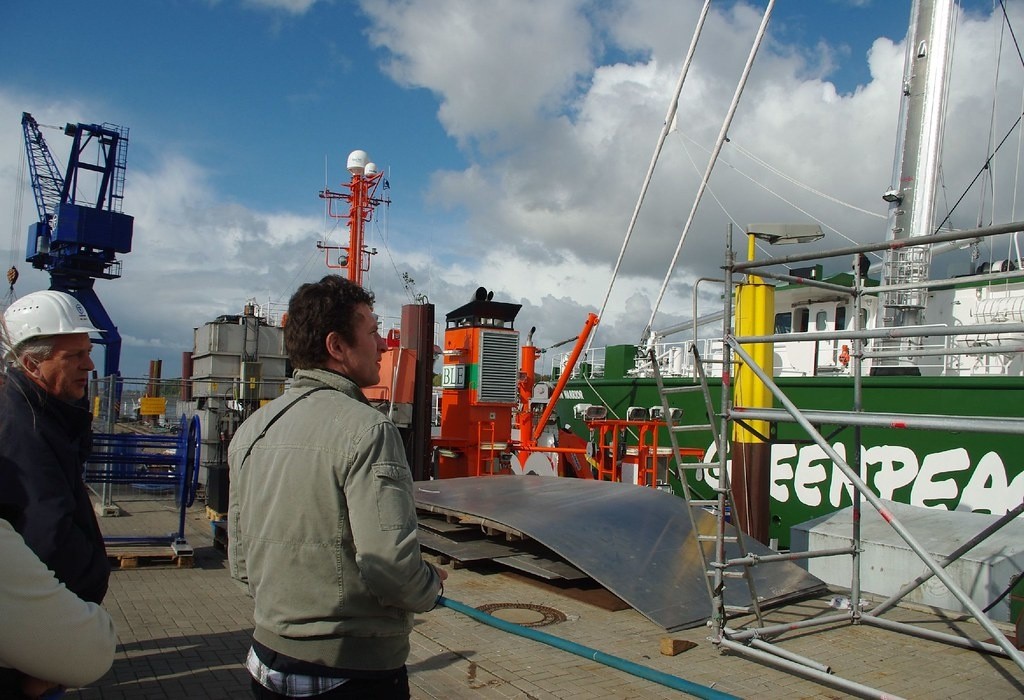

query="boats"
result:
[532,0,1022,554]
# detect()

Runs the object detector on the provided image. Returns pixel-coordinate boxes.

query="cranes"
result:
[6,109,137,420]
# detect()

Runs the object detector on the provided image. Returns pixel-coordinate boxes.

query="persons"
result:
[226,275,447,700]
[0,290,116,700]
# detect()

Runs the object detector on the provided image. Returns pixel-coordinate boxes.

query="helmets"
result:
[0,290,108,363]
